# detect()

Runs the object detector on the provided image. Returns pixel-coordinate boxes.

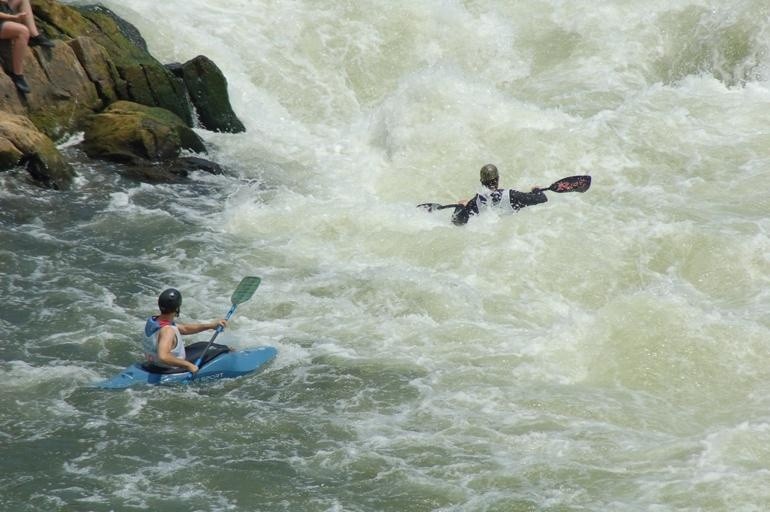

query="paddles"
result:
[187,276,261,380]
[417,175,591,212]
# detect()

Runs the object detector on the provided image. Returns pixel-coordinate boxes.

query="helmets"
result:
[479,164,498,183]
[158,288,182,314]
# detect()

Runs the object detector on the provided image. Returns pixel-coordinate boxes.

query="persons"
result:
[451,164,548,226]
[143,288,229,374]
[0,0,56,93]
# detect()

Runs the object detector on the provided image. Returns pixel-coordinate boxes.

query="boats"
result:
[89,346,280,392]
